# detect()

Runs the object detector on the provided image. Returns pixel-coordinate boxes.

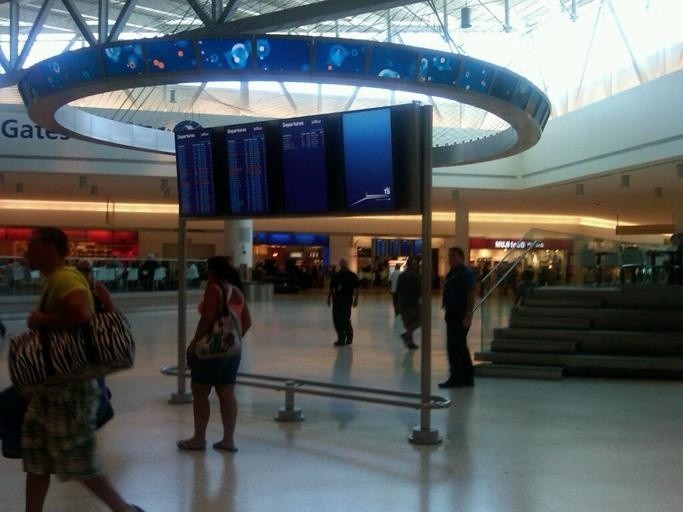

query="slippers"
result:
[177,440,205,450]
[213,441,237,451]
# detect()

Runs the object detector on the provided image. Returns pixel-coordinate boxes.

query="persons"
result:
[75,257,117,432]
[513,280,525,306]
[387,264,400,315]
[397,256,421,351]
[326,257,358,346]
[436,247,475,388]
[22,226,147,511]
[177,255,251,451]
[2,249,682,295]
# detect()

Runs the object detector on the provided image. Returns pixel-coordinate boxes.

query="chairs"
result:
[9,262,204,296]
[576,241,677,286]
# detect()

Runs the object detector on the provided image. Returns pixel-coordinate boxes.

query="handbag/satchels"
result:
[8,313,135,385]
[0,375,114,458]
[195,315,242,359]
